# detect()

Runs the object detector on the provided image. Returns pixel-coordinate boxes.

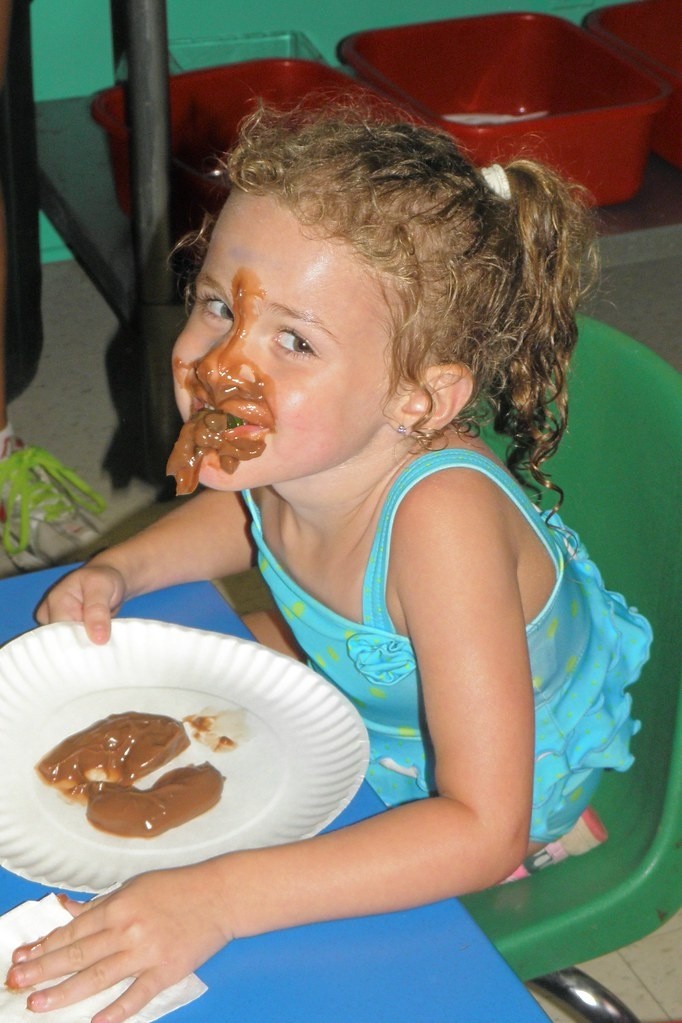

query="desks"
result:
[0,560,551,1023]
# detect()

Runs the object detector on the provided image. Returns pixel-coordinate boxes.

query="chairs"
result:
[450,311,682,1023]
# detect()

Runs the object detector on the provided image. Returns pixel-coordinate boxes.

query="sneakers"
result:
[0,445,110,572]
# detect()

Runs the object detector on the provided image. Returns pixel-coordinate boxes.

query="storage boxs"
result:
[89,0,682,248]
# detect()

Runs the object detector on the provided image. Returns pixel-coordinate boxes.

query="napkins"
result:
[0,881,210,1023]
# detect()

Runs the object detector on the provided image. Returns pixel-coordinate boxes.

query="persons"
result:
[6,83,654,1021]
